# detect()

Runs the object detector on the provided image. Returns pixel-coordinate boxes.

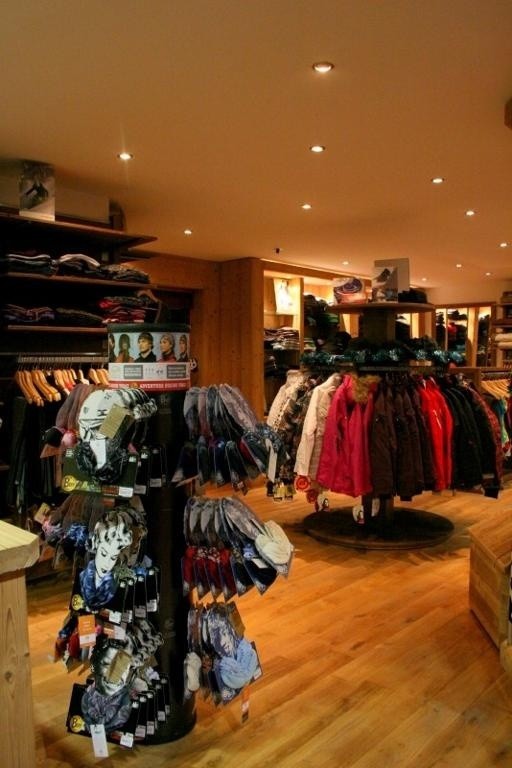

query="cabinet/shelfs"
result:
[393,311,412,342]
[3,211,160,336]
[446,308,467,366]
[435,306,447,351]
[474,305,491,367]
[302,275,350,357]
[216,259,303,425]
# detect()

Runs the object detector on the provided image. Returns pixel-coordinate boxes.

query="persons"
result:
[133,331,156,363]
[108,334,116,363]
[156,332,176,363]
[113,334,134,363]
[83,638,134,727]
[208,607,259,692]
[79,526,131,609]
[176,335,188,362]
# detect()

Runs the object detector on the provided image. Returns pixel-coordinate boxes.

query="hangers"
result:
[288,361,511,404]
[11,354,112,410]
